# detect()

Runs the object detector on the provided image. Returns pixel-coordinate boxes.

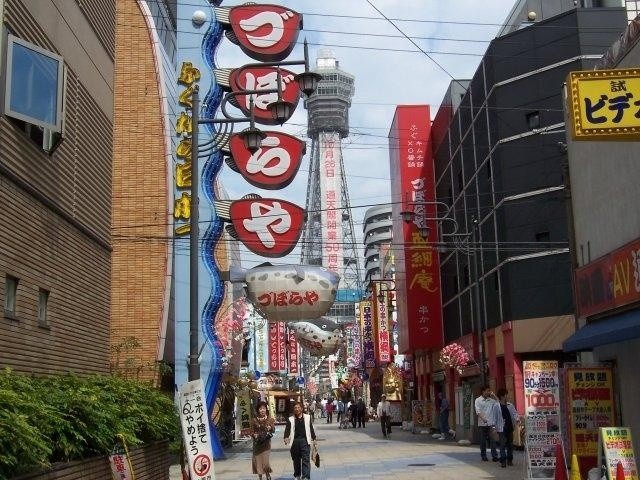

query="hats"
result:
[381,394,386,398]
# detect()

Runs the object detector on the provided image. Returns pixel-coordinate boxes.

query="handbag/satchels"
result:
[311,447,320,467]
[254,432,272,446]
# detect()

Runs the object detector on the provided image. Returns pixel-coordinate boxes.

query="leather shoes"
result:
[502,461,506,468]
[493,457,498,462]
[507,459,513,466]
[482,457,489,461]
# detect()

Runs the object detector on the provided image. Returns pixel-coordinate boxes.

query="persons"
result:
[437,393,456,440]
[475,385,499,462]
[493,388,521,467]
[377,394,393,440]
[250,401,275,480]
[283,402,317,480]
[302,395,374,429]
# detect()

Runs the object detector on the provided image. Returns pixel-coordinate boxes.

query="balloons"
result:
[245,264,340,321]
[285,318,343,357]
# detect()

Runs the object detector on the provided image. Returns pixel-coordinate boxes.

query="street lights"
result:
[401,193,488,396]
[186,39,321,380]
